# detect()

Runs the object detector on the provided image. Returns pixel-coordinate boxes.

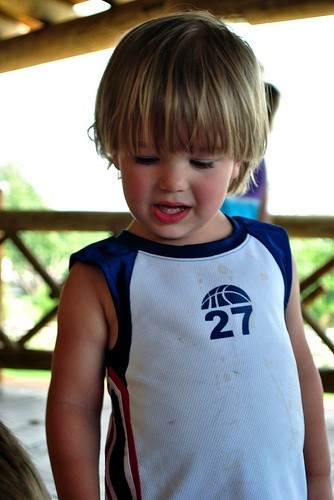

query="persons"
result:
[218,81,282,226]
[0,422,58,500]
[46,10,331,500]
